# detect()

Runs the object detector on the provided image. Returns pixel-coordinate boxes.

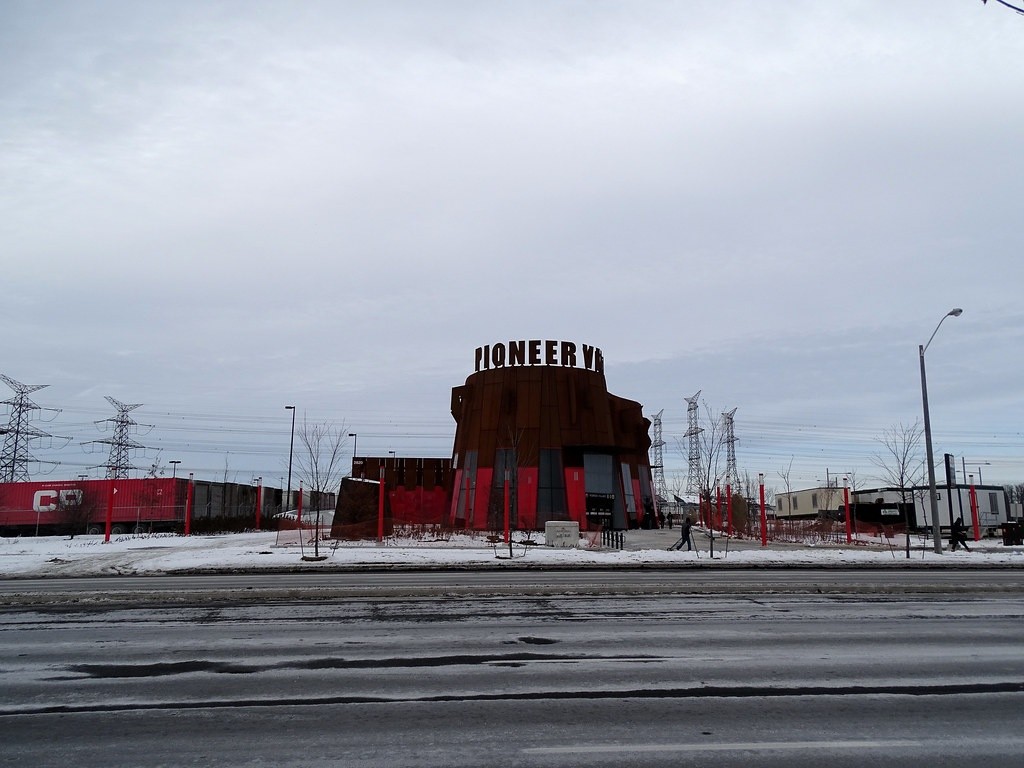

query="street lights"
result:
[349,434,357,457]
[285,406,296,512]
[956,467,982,488]
[962,456,991,485]
[389,451,396,472]
[169,461,181,478]
[827,468,852,487]
[919,307,963,556]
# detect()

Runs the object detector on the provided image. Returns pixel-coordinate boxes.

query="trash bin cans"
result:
[1002,523,1019,546]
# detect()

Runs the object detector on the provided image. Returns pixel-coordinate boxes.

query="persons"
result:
[659,512,665,529]
[951,517,973,552]
[677,517,691,551]
[667,512,673,529]
